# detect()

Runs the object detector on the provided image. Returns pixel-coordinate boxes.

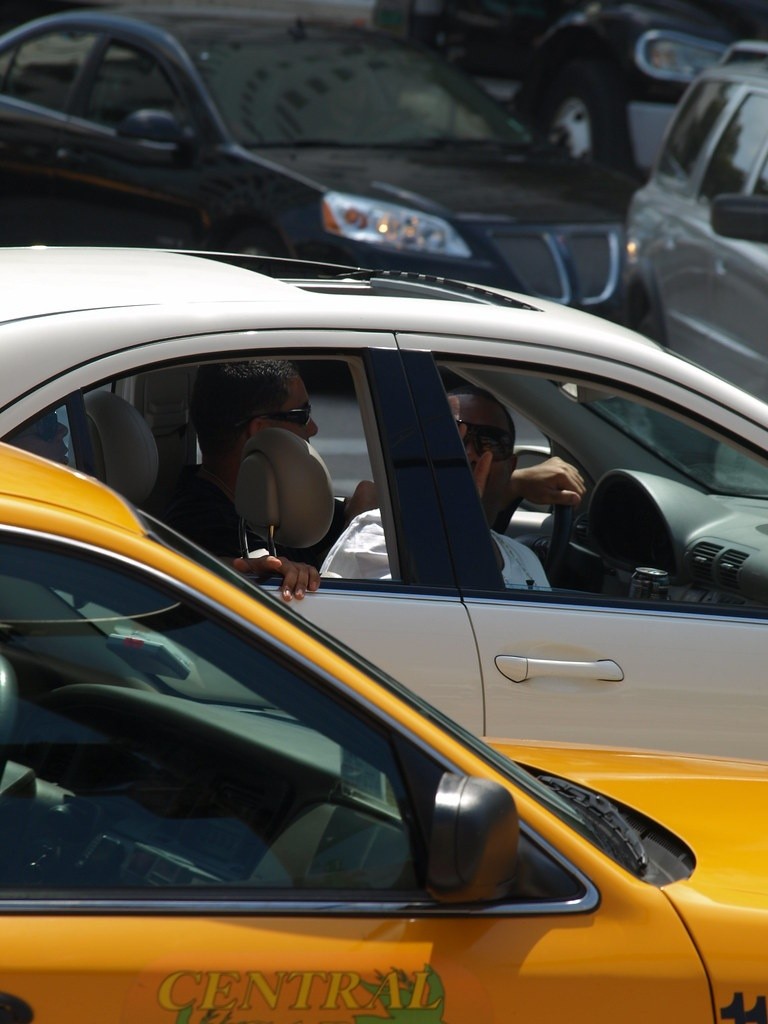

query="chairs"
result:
[232,427,334,570]
[84,390,158,504]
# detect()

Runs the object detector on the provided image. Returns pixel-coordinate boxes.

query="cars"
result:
[385,0,765,204]
[0,244,766,758]
[2,444,768,1024]
[0,1,671,348]
[615,41,768,426]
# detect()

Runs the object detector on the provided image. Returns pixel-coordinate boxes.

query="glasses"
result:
[455,420,513,462]
[235,400,311,427]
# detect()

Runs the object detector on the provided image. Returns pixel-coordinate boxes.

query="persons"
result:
[157,360,587,602]
